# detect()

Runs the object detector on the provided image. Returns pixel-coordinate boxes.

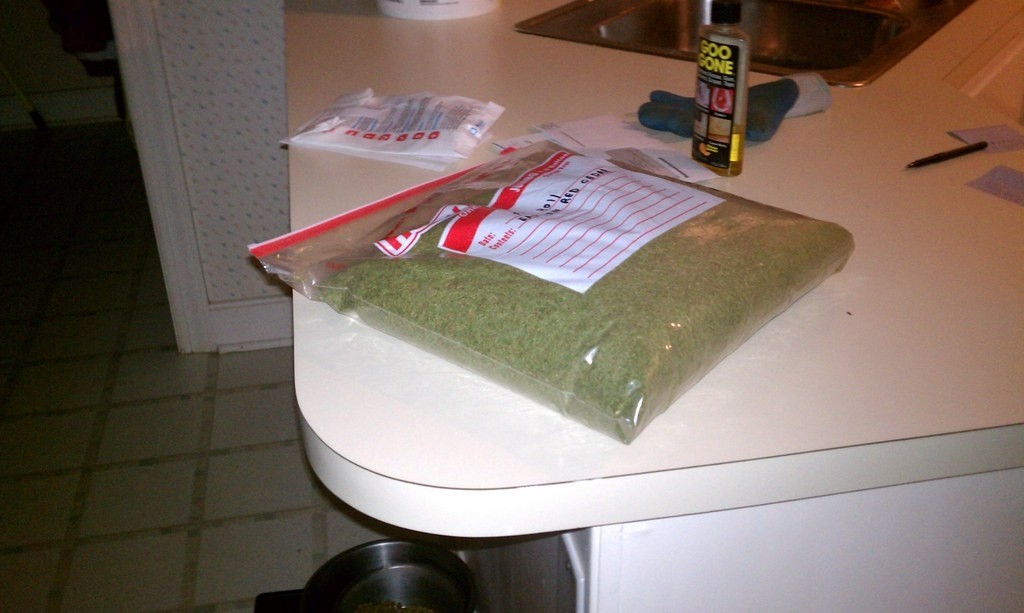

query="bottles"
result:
[692,1,751,177]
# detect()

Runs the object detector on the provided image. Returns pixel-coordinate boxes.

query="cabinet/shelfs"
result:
[469,468,1024,613]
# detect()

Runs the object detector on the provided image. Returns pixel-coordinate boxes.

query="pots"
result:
[253,539,477,613]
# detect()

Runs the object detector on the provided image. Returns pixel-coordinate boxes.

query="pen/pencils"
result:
[904,140,988,169]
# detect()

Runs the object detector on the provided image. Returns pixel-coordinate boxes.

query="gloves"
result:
[638,72,832,142]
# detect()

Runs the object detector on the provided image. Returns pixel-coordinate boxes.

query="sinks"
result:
[587,0,913,89]
